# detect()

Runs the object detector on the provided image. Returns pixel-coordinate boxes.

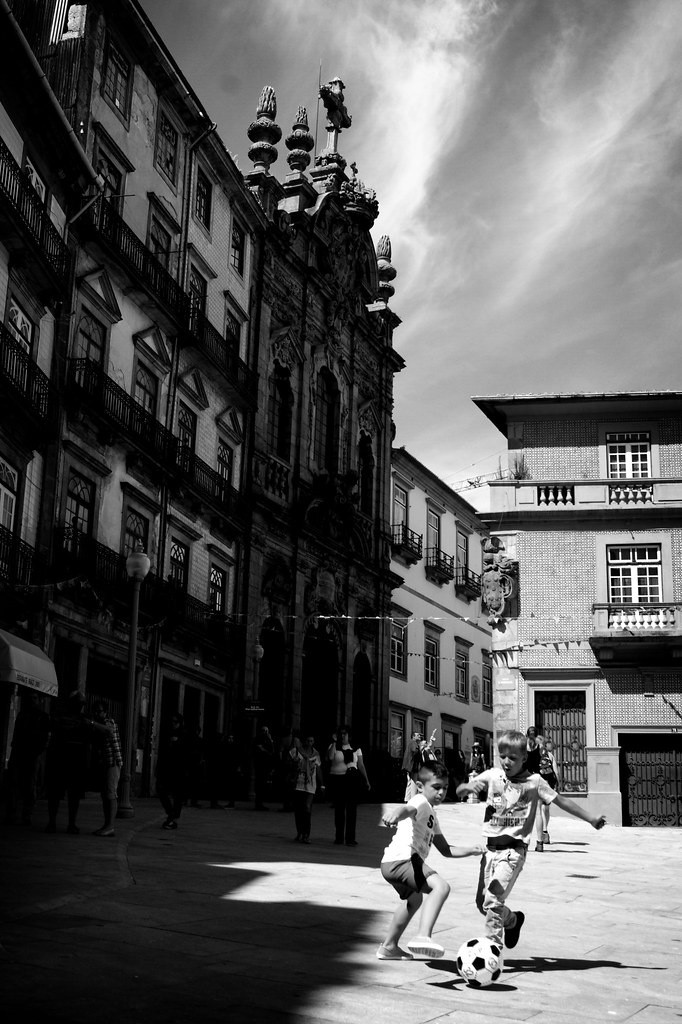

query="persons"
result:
[2,691,123,836]
[527,727,560,852]
[158,713,296,829]
[376,760,488,960]
[456,730,607,950]
[328,725,371,847]
[453,742,487,802]
[289,734,322,844]
[401,728,438,801]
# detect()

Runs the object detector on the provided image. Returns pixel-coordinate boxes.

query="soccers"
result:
[456,937,503,986]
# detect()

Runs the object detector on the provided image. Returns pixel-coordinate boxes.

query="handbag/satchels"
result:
[346,768,364,789]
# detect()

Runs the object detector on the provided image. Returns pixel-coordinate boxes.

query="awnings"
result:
[0,629,58,698]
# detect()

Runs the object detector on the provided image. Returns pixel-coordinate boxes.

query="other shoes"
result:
[294,834,304,842]
[535,840,545,852]
[503,909,525,949]
[334,839,345,845]
[542,833,551,844]
[348,837,359,846]
[163,819,177,830]
[377,943,413,961]
[302,836,313,843]
[408,937,451,958]
[95,828,116,836]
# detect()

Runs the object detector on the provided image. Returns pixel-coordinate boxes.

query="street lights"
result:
[245,635,266,801]
[111,537,153,821]
